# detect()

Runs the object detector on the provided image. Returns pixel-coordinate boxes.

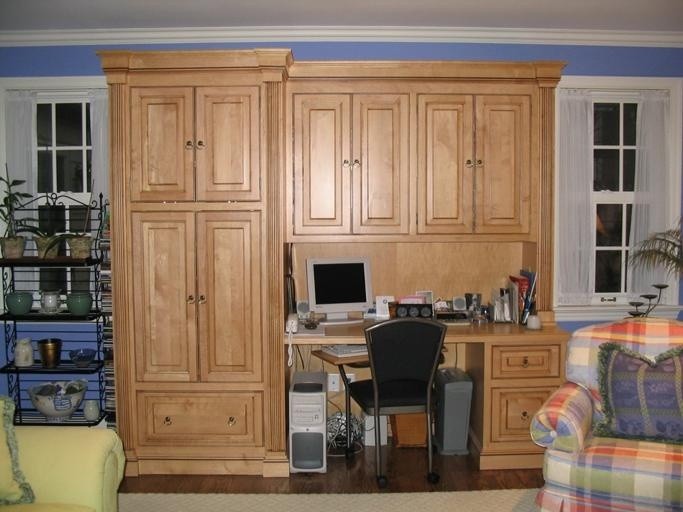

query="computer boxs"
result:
[289,371,328,474]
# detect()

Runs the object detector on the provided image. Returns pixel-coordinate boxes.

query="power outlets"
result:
[341,374,355,389]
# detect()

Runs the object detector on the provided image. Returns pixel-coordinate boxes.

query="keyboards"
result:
[322,345,369,358]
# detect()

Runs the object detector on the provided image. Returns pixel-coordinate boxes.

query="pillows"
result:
[595,342,683,447]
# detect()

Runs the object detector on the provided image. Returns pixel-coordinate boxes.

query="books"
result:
[509,274,530,323]
[99,216,116,429]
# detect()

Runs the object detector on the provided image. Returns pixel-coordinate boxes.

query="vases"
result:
[70,233,93,261]
[34,235,59,259]
[5,291,34,315]
[67,291,92,318]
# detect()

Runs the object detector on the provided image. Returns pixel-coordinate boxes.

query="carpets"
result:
[119,486,543,512]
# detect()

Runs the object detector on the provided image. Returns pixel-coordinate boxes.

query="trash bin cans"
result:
[433,368,473,455]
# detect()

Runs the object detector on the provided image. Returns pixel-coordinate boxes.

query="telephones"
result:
[285,312,299,334]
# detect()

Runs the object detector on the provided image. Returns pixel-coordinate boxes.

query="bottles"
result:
[14,337,34,368]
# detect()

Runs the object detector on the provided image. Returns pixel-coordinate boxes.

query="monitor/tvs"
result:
[306,258,373,324]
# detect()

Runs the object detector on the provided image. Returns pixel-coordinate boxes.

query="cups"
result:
[527,315,542,330]
[83,400,100,421]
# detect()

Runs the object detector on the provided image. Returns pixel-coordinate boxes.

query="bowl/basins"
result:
[69,349,96,368]
[27,380,88,422]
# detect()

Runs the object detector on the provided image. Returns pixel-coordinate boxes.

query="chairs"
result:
[530,317,683,512]
[342,317,450,488]
[0,398,125,512]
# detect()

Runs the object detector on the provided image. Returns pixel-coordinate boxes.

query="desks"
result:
[284,320,572,472]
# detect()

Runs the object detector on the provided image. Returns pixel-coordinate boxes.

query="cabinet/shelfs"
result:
[110,204,289,477]
[97,48,293,203]
[285,60,414,243]
[0,191,113,428]
[415,60,569,243]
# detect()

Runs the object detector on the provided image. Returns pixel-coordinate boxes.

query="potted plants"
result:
[0,163,30,259]
